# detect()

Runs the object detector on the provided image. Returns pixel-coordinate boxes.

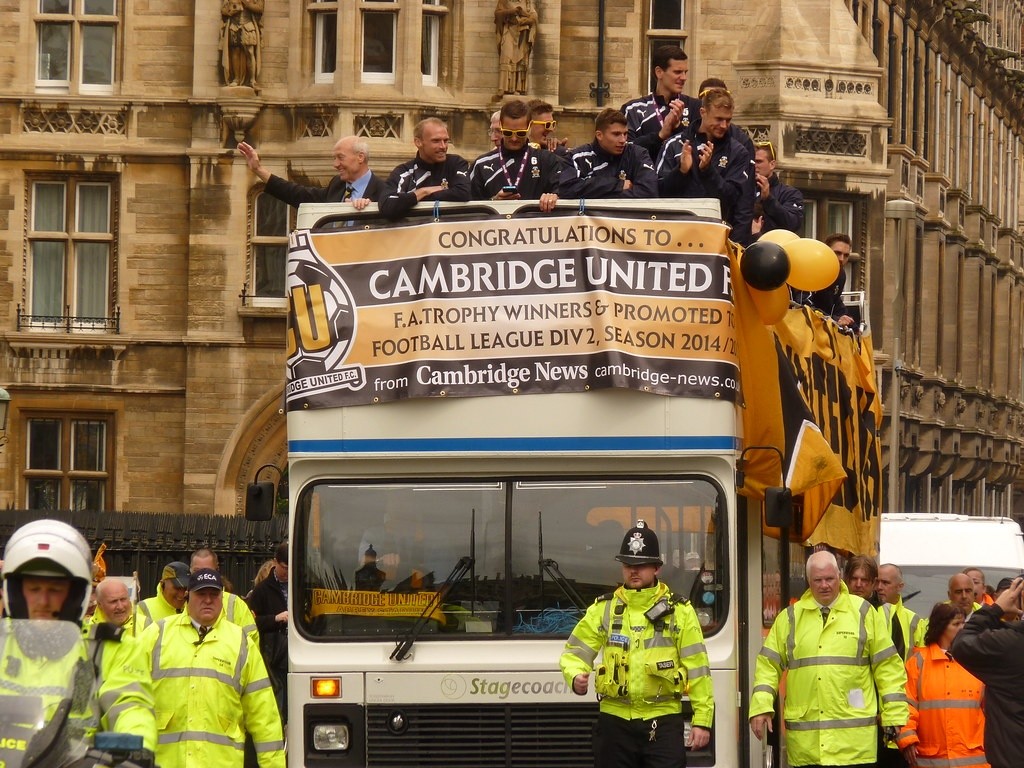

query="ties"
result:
[329,186,355,231]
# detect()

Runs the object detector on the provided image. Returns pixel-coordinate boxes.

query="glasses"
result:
[699,88,731,100]
[532,120,557,129]
[500,124,532,137]
[754,141,776,161]
[277,563,290,570]
[486,128,500,137]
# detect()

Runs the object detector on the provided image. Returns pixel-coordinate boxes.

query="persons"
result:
[83,577,158,641]
[494,0,539,97]
[244,559,276,599]
[991,577,1023,626]
[920,572,986,648]
[187,548,261,652]
[962,567,997,612]
[243,541,289,724]
[293,478,796,652]
[842,554,907,768]
[949,576,1024,768]
[875,562,925,768]
[132,560,193,627]
[746,549,910,768]
[132,567,288,768]
[0,516,159,768]
[220,0,266,92]
[236,43,876,339]
[893,603,993,768]
[558,518,716,768]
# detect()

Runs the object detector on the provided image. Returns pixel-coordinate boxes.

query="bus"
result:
[283,196,885,765]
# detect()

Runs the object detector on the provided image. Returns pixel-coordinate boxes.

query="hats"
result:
[614,519,663,566]
[161,562,193,592]
[993,576,1014,591]
[188,567,222,590]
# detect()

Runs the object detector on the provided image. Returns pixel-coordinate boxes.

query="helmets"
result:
[2,520,93,621]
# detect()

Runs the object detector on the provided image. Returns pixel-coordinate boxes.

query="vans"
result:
[867,511,1023,625]
